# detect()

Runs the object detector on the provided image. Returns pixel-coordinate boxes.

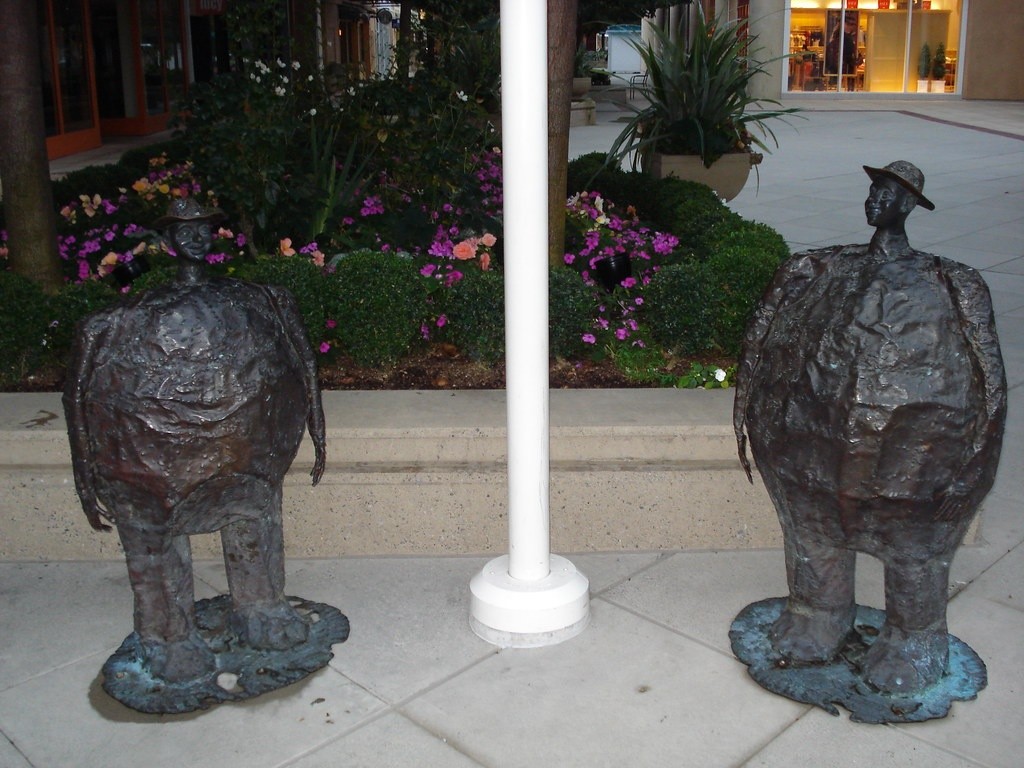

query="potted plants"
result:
[580,0,808,206]
[916,39,932,93]
[931,41,947,94]
[572,42,597,101]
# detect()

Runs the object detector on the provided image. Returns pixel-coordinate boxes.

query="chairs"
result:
[629,69,650,99]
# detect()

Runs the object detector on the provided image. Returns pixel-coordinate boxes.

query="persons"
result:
[846,74,860,94]
[60,195,352,716]
[827,14,857,74]
[729,159,986,724]
